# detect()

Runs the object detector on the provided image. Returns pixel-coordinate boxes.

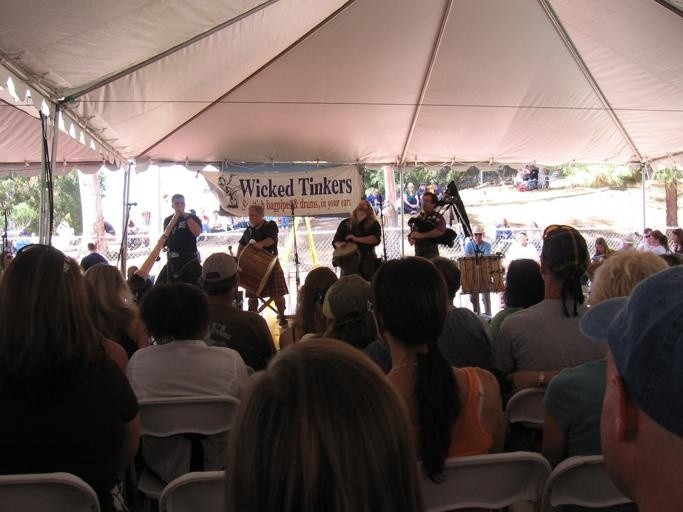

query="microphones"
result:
[444,195,455,204]
[228,246,232,254]
[128,202,138,206]
[291,203,294,211]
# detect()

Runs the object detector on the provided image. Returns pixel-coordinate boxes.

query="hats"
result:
[418,180,426,184]
[472,226,484,233]
[679,229,682,245]
[201,253,238,282]
[578,265,682,438]
[322,273,372,323]
[621,232,637,244]
[407,182,413,186]
[543,227,589,279]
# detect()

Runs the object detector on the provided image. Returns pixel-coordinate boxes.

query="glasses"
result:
[644,235,649,237]
[6,255,12,259]
[543,225,574,240]
[88,243,96,250]
[474,234,481,236]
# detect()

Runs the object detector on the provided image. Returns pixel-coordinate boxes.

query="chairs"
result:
[505,387,547,452]
[136,396,242,502]
[418,451,552,512]
[542,455,639,512]
[158,470,225,512]
[0,471,101,512]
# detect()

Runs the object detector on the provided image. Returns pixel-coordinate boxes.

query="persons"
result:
[124,282,249,486]
[161,193,203,282]
[237,205,290,326]
[579,263,683,512]
[509,231,540,263]
[671,228,683,253]
[199,252,278,373]
[542,247,670,460]
[367,188,382,214]
[402,182,420,215]
[650,230,671,255]
[140,210,160,261]
[81,275,128,373]
[208,209,225,233]
[299,274,378,350]
[417,182,428,210]
[433,182,442,196]
[367,256,506,482]
[81,243,109,273]
[407,192,447,260]
[0,243,142,512]
[491,258,545,337]
[637,228,653,252]
[332,198,382,278]
[0,250,14,281]
[363,255,493,375]
[59,211,73,228]
[84,262,150,361]
[223,336,423,512]
[278,267,339,348]
[619,234,635,249]
[463,225,491,316]
[491,224,607,390]
[590,237,609,264]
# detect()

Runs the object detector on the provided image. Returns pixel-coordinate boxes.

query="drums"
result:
[237,239,277,296]
[333,243,361,274]
[458,252,505,294]
